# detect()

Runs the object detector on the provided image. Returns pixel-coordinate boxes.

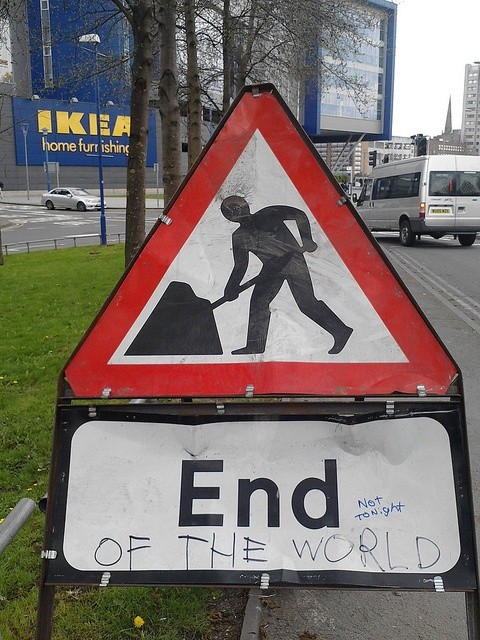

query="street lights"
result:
[41,127,51,192]
[20,123,30,200]
[79,33,107,245]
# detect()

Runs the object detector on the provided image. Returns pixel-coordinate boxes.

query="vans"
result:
[352,155,480,246]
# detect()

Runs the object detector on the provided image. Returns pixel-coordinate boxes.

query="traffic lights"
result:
[417,137,426,155]
[369,150,377,167]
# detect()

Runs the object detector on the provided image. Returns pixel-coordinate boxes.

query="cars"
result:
[41,187,107,212]
